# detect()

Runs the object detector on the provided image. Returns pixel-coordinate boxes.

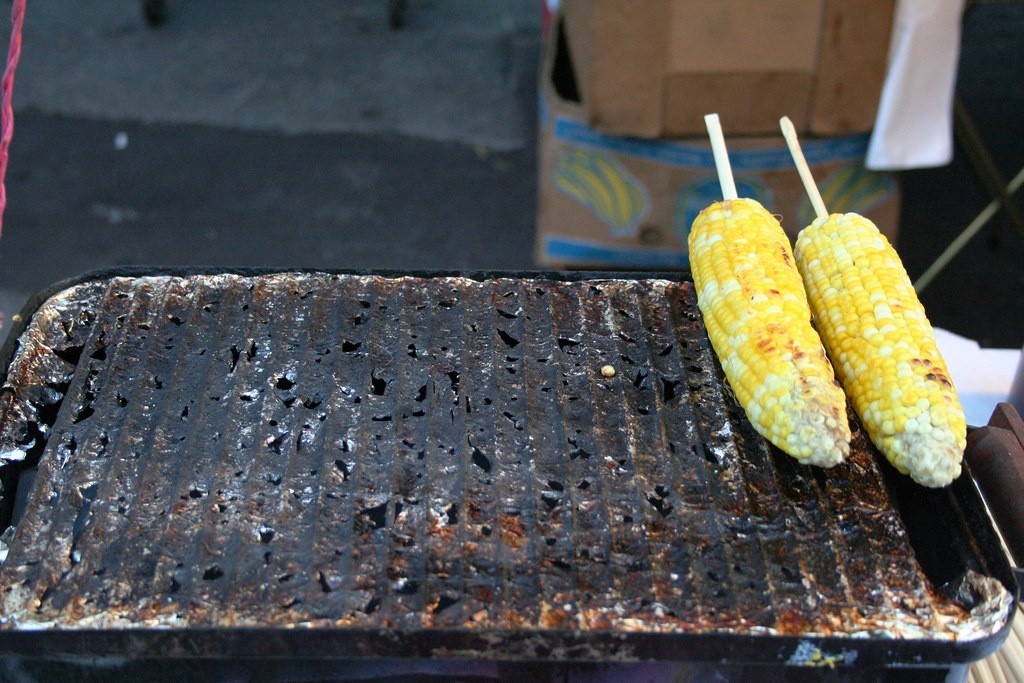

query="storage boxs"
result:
[533,0,903,272]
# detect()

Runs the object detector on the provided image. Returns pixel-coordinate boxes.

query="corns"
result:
[793,213,967,488]
[687,198,852,468]
